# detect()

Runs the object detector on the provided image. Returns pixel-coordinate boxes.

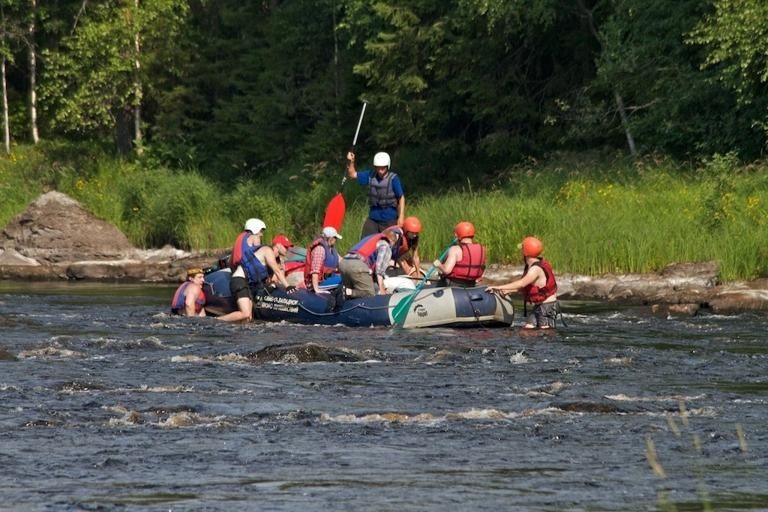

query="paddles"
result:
[322,102,366,232]
[391,237,458,325]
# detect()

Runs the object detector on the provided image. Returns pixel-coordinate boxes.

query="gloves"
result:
[285,286,296,292]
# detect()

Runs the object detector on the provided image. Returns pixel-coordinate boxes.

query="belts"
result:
[343,257,358,259]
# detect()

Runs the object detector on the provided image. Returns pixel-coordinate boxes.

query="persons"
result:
[168,267,207,317]
[345,150,406,239]
[432,221,488,287]
[484,236,558,328]
[213,215,432,322]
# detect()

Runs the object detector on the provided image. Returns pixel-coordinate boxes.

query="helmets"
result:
[522,236,544,257]
[244,218,266,235]
[454,222,474,240]
[373,152,391,170]
[403,217,421,235]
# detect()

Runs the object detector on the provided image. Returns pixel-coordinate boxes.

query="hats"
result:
[322,226,343,239]
[272,235,294,247]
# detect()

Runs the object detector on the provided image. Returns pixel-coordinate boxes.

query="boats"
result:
[196,246,516,350]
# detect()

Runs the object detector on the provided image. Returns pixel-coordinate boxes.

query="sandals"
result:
[336,284,345,307]
[328,289,336,312]
